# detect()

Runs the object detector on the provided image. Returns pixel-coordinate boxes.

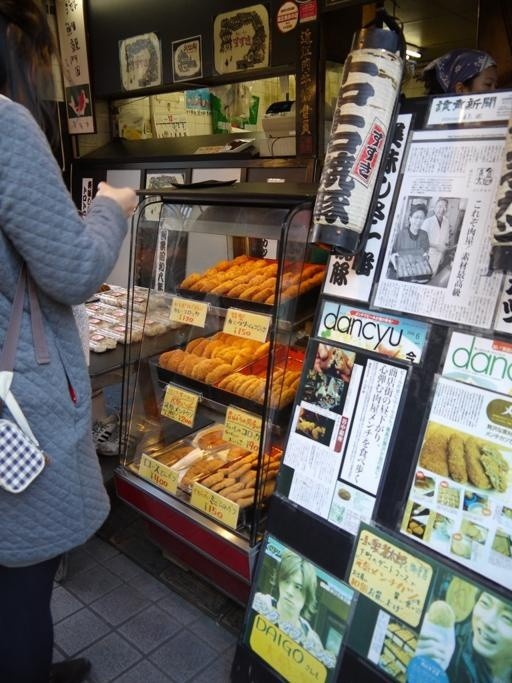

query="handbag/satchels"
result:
[0,419,48,492]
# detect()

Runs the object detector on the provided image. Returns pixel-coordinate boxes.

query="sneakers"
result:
[53,658,91,682]
[92,411,138,456]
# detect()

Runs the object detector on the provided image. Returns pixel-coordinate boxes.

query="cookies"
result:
[202,454,279,506]
[159,332,301,408]
[421,432,508,492]
[181,255,326,303]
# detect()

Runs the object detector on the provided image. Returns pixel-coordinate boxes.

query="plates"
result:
[416,488,433,496]
[473,526,488,544]
[220,137,258,152]
[451,538,470,559]
[170,179,238,190]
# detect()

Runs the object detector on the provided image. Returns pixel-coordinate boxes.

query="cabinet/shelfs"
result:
[109,186,318,609]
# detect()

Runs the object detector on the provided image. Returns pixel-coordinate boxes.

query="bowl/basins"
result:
[465,493,486,513]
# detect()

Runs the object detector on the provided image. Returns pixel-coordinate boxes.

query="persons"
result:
[251,552,324,658]
[421,197,451,280]
[390,203,428,270]
[0,0,138,682]
[422,47,499,94]
[447,588,511,682]
